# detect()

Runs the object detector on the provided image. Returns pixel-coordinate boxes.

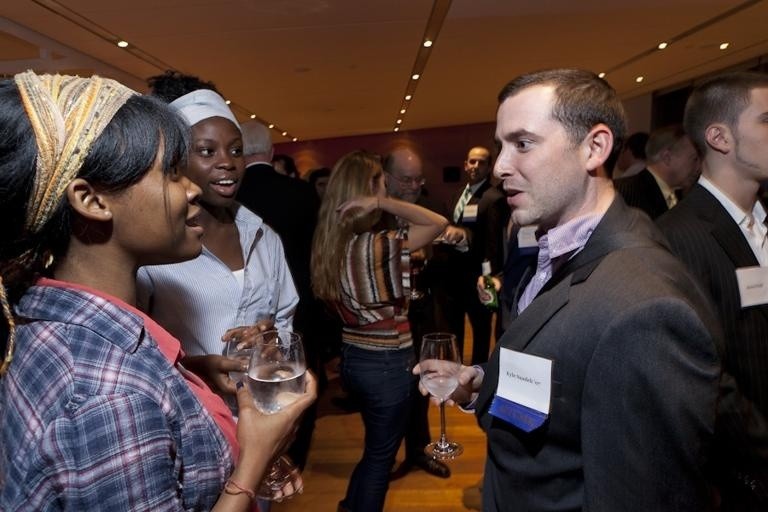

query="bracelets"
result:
[222,473,257,503]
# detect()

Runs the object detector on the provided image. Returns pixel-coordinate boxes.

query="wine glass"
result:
[246,330,305,498]
[410,245,428,299]
[227,342,250,384]
[420,333,464,460]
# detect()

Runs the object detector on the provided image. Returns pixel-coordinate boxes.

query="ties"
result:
[453,185,471,224]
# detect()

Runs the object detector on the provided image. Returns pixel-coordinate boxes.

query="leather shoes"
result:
[413,453,450,477]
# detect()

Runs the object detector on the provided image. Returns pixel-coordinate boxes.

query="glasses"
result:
[389,173,426,186]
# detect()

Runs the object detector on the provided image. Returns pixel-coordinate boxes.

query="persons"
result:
[137,68,302,511]
[310,149,450,512]
[384,147,539,481]
[650,69,766,511]
[0,69,319,511]
[411,69,727,512]
[613,127,704,222]
[237,122,335,475]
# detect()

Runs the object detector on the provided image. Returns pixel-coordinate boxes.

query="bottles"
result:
[480,258,502,313]
